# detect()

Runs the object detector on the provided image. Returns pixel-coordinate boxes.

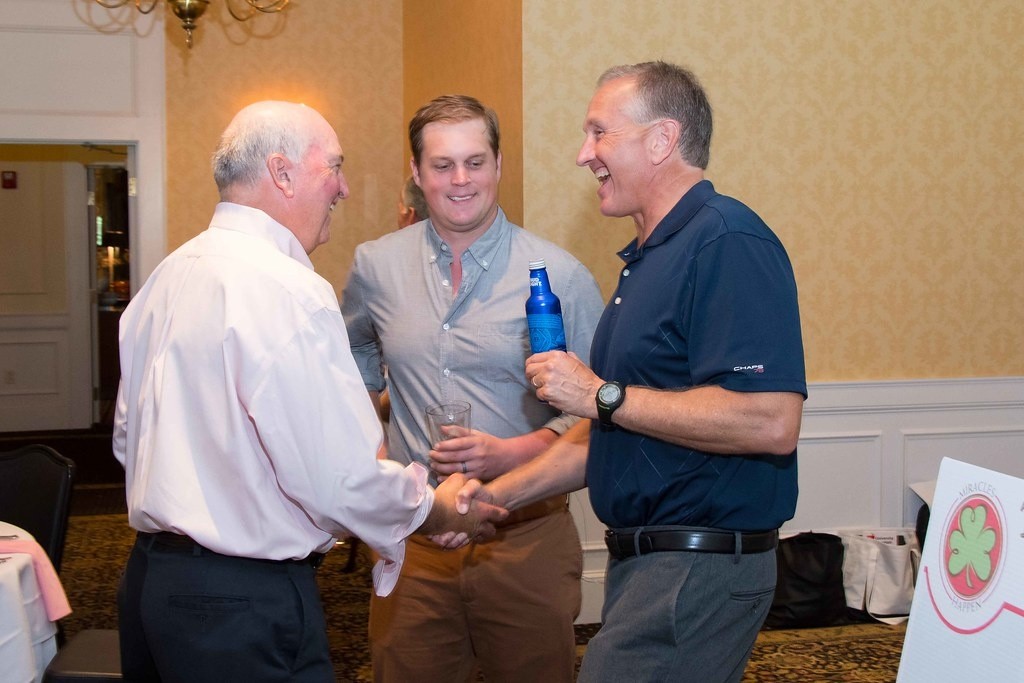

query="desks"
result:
[0,521,59,683]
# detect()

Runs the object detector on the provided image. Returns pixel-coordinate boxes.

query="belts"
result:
[138,531,208,552]
[602,528,779,555]
[492,493,568,526]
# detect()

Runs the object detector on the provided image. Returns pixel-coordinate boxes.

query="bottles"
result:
[526,258,567,404]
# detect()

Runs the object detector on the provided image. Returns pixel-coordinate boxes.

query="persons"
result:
[112,102,510,683]
[341,95,606,682]
[427,60,808,682]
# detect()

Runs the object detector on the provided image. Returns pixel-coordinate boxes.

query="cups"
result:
[425,400,471,476]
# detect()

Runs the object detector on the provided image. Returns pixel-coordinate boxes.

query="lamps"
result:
[96,0,291,49]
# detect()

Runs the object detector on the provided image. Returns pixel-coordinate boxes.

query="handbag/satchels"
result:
[761,530,917,631]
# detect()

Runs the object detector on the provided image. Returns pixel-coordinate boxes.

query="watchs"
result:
[596,380,627,430]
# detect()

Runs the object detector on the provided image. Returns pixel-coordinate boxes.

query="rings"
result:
[531,376,540,388]
[462,461,466,472]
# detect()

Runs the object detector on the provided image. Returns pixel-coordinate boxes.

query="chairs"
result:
[0,443,78,647]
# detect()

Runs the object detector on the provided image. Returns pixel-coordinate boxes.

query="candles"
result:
[108,246,114,283]
